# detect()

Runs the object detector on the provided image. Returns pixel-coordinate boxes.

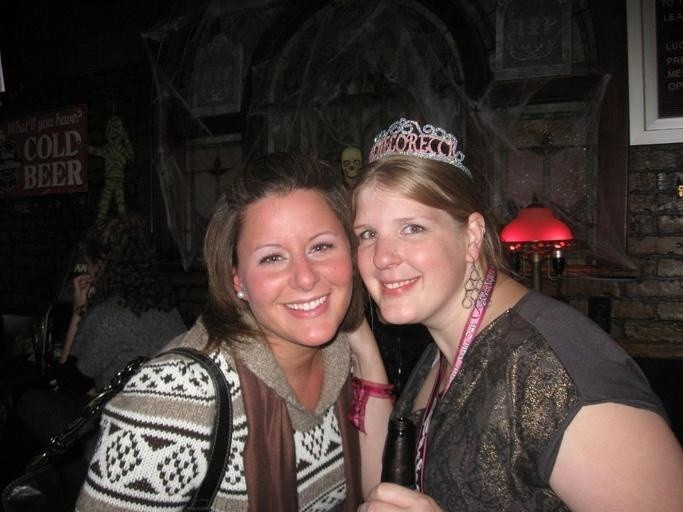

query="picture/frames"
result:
[186,41,249,254]
[626,0,683,150]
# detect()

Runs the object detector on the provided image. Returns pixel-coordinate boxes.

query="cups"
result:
[30,323,55,373]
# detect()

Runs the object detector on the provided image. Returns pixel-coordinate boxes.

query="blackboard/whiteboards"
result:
[1,67,150,319]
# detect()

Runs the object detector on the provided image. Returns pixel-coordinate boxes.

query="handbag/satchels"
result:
[0,346,234,512]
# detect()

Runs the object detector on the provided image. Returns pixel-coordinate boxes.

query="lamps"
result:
[499,190,571,294]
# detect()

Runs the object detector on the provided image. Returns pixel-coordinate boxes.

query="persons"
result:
[56,212,191,396]
[71,140,396,512]
[76,114,136,232]
[355,114,683,512]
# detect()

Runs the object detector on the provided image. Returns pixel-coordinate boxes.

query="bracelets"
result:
[349,373,395,402]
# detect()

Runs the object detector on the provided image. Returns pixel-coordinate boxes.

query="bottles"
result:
[380,417,419,493]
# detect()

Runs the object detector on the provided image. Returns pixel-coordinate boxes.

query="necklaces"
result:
[410,257,497,498]
